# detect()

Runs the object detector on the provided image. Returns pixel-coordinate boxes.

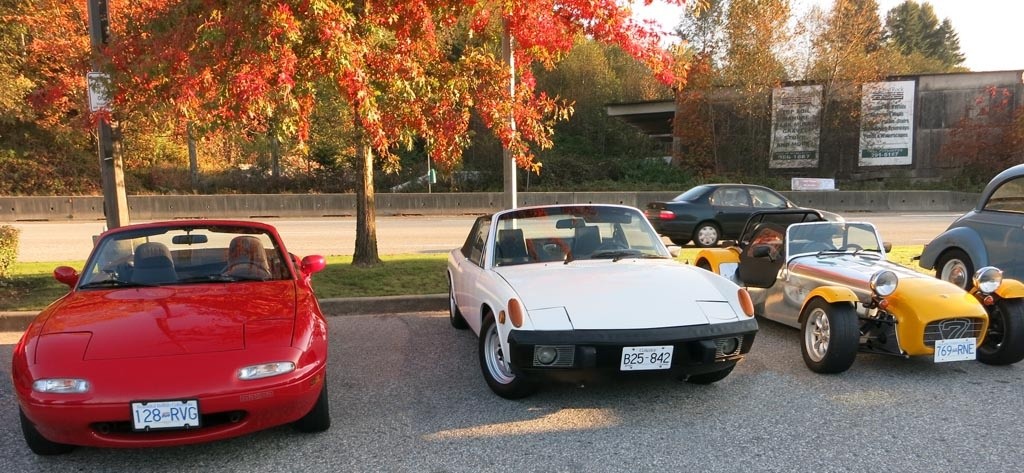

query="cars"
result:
[642,184,847,249]
[911,161,1024,294]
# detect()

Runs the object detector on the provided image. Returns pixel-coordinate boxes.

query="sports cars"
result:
[445,203,761,402]
[669,209,1024,374]
[11,219,331,456]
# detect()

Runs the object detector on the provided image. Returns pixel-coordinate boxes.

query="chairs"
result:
[801,241,833,252]
[222,236,270,276]
[131,242,178,283]
[572,226,602,258]
[496,228,530,257]
[748,243,780,258]
